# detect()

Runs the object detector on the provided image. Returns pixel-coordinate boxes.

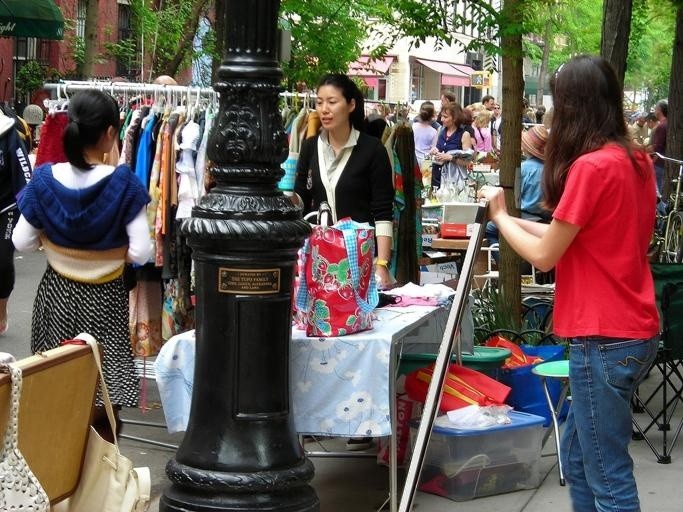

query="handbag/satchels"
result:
[69,335,150,511]
[292,217,379,337]
[1,353,50,512]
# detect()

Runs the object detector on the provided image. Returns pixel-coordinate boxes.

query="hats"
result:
[521,125,549,162]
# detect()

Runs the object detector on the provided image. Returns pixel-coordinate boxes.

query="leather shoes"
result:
[1,321,8,335]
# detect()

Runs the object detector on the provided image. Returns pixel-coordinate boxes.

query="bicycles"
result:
[650,151,683,264]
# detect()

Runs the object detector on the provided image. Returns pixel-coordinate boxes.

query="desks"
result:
[154,294,459,512]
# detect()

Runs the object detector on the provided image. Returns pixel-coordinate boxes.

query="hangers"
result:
[48,80,317,142]
[362,98,413,120]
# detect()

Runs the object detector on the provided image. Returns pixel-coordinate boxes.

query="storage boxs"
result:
[409,411,549,505]
[397,342,512,400]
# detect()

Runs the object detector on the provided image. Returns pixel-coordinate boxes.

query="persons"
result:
[646,99,669,239]
[430,102,472,191]
[491,103,502,151]
[485,124,549,266]
[436,91,456,125]
[475,54,661,512]
[645,112,659,130]
[535,106,546,124]
[0,101,35,334]
[460,108,478,151]
[10,88,158,446]
[412,101,442,176]
[632,111,648,145]
[482,95,497,150]
[414,102,442,131]
[287,71,396,293]
[471,110,492,152]
[522,97,536,123]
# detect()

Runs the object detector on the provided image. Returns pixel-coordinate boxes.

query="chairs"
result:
[530,358,574,489]
[624,280,683,467]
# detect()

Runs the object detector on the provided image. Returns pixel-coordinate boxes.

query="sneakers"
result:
[347,435,373,450]
[299,434,321,443]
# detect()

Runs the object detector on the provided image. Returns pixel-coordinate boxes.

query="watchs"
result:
[376,260,391,269]
